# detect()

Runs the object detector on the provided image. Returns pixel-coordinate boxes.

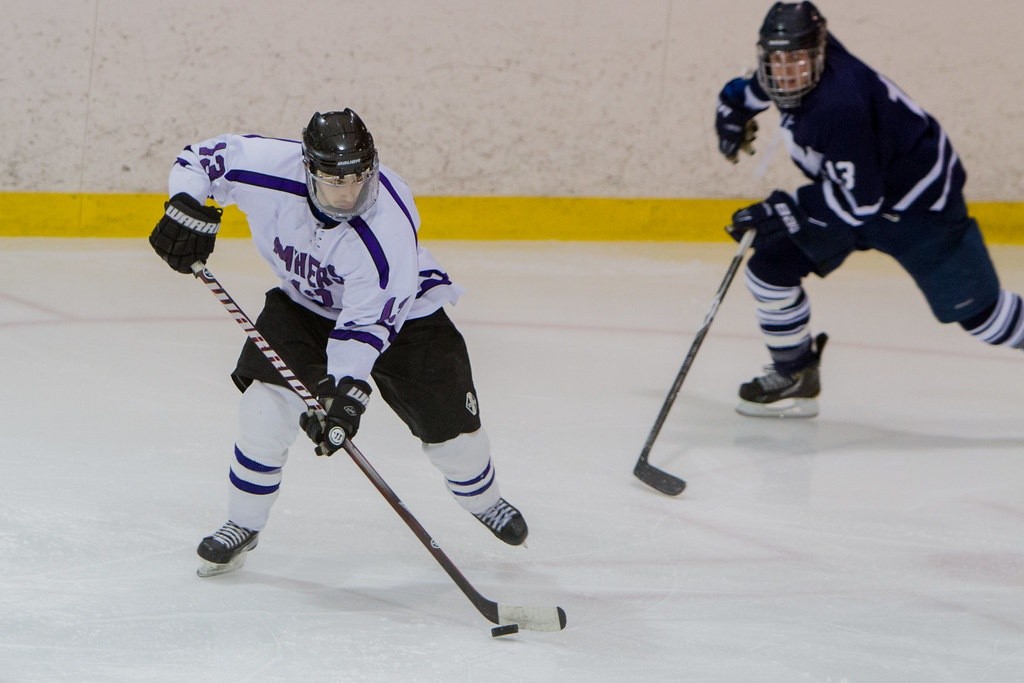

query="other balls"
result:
[489,622,521,637]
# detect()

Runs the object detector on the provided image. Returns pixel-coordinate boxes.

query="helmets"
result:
[757,0,827,108]
[303,109,380,222]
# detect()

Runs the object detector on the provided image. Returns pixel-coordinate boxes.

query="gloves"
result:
[715,100,759,164]
[299,373,372,457]
[146,191,223,274]
[724,191,802,251]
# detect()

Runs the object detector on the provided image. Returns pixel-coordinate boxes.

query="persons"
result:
[715,0,1024,417]
[145,107,529,577]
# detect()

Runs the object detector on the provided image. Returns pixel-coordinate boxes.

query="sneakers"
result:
[196,520,258,577]
[736,332,828,418]
[470,497,529,550]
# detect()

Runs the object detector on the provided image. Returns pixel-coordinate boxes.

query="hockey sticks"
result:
[189,259,569,636]
[628,228,758,499]
